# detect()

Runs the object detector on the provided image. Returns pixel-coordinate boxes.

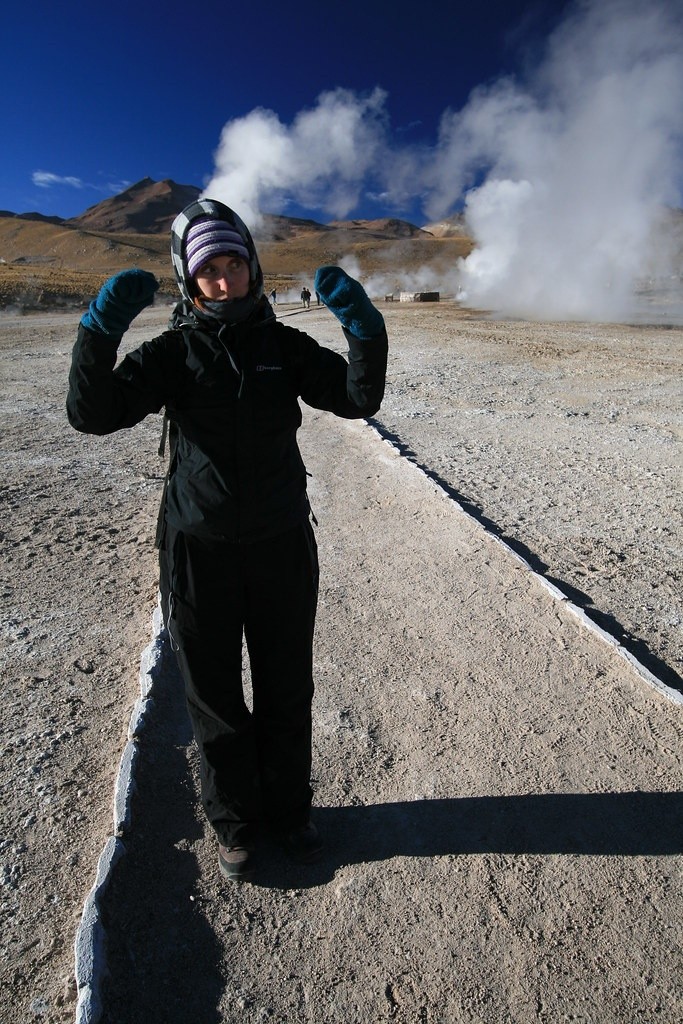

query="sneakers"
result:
[273,819,325,864]
[218,839,258,880]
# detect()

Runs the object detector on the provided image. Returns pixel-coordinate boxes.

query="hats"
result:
[183,215,251,275]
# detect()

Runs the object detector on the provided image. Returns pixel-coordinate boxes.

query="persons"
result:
[301,287,311,308]
[67,199,389,874]
[269,288,277,305]
[315,291,320,305]
